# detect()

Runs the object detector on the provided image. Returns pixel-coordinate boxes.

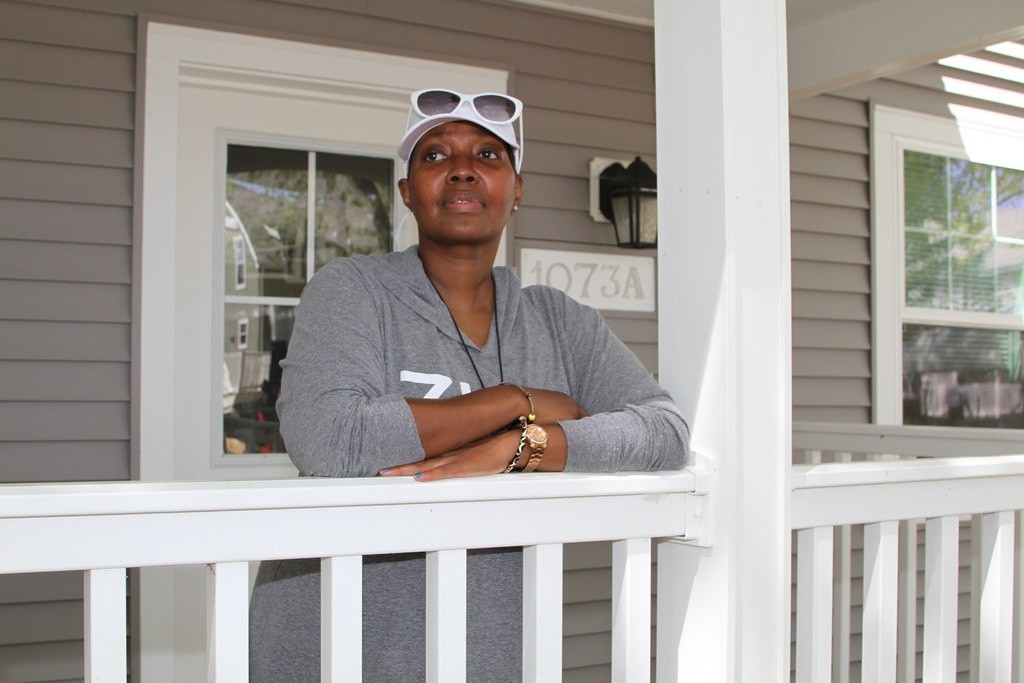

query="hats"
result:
[396,85,525,178]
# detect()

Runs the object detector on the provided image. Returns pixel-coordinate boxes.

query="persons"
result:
[249,88,690,683]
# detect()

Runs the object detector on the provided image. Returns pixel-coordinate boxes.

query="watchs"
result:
[519,424,548,472]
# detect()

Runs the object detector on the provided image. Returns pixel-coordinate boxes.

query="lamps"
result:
[589,157,658,249]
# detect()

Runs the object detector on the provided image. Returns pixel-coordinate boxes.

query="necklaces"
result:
[425,272,504,390]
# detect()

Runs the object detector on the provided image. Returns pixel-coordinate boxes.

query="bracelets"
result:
[499,381,536,424]
[503,416,527,473]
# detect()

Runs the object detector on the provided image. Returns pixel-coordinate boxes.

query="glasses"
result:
[405,88,523,174]
